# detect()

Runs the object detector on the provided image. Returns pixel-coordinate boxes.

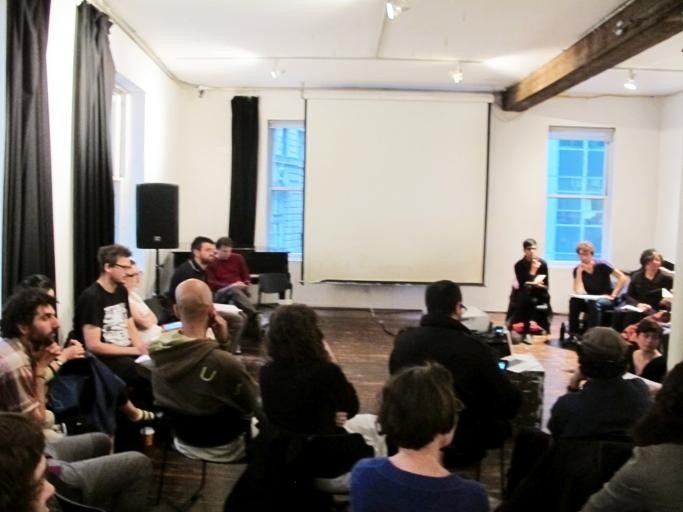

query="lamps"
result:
[623,68,638,92]
[383,1,407,20]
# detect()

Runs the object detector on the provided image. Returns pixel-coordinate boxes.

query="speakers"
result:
[136,185,179,248]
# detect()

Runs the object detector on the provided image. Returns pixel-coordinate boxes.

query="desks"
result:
[500,344,662,438]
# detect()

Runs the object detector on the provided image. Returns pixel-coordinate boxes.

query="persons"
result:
[349,360,489,512]
[563,240,627,349]
[505,237,554,335]
[168,235,249,354]
[622,320,666,389]
[12,273,162,446]
[76,244,159,405]
[613,250,672,332]
[0,289,155,512]
[502,325,652,497]
[580,362,683,512]
[133,277,263,463]
[256,304,389,495]
[221,413,337,512]
[206,236,268,333]
[389,279,520,469]
[2,410,57,512]
[121,260,165,345]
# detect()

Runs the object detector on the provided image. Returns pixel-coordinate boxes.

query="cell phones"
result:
[497,359,508,371]
[494,326,503,334]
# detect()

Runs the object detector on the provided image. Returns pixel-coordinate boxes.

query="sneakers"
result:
[130,407,163,421]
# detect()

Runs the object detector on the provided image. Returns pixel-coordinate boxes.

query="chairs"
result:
[254,272,293,312]
[143,295,173,327]
[386,409,505,497]
[153,393,253,505]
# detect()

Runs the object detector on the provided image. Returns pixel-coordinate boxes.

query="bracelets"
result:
[34,374,46,380]
[54,358,63,368]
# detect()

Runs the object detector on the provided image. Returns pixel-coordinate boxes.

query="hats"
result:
[581,326,627,364]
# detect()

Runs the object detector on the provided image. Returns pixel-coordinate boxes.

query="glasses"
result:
[459,304,466,313]
[126,271,142,277]
[114,264,131,270]
